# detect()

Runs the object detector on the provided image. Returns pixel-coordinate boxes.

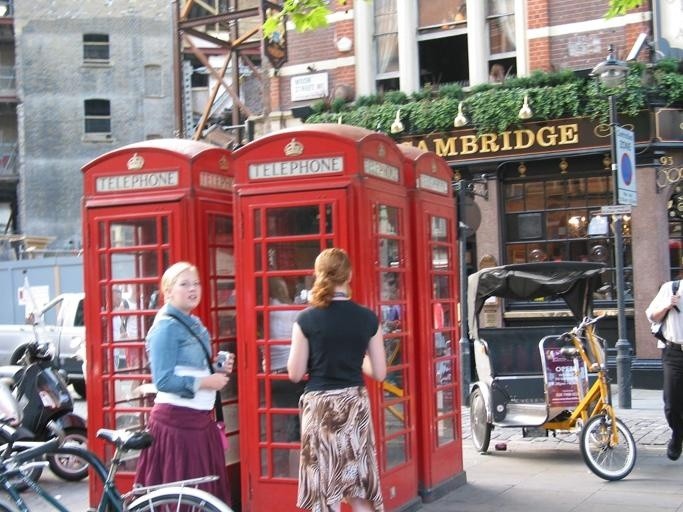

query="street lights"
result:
[589,41,638,412]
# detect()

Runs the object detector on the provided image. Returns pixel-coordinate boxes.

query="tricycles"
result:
[466,258,639,483]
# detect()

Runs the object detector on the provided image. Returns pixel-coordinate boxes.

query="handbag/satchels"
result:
[650,278,680,345]
[215,419,230,452]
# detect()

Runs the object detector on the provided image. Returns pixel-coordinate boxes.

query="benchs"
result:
[474,328,606,397]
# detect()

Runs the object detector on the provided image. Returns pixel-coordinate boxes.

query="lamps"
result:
[455,100,471,129]
[518,94,534,121]
[388,110,405,133]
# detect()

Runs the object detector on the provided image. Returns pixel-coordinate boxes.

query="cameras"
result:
[214,351,230,373]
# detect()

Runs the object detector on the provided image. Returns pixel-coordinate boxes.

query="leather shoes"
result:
[666,437,683,461]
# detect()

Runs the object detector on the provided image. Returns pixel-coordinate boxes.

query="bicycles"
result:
[1,423,236,512]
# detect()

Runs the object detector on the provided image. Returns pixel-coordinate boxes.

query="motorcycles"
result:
[1,339,94,483]
[0,375,48,496]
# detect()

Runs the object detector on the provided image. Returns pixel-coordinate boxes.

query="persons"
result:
[285,247,390,512]
[642,277,683,462]
[129,260,236,512]
[103,288,129,370]
[256,277,314,442]
[489,63,506,83]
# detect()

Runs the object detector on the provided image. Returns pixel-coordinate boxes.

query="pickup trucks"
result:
[0,291,131,400]
